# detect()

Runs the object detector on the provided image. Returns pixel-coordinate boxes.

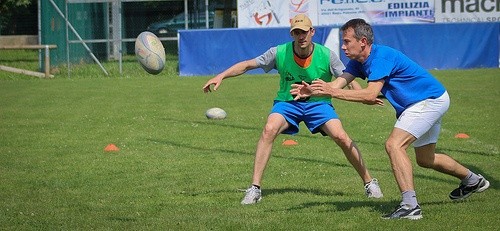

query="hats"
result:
[289,13,313,32]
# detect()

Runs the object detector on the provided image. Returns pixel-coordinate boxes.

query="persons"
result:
[289,18,491,221]
[202,14,384,207]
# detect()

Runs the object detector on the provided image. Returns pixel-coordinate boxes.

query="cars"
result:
[146,11,214,37]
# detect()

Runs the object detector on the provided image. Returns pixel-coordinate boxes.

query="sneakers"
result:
[241,185,262,204]
[449,174,489,199]
[364,178,384,199]
[380,204,424,221]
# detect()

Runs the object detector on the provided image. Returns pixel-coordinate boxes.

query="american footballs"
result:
[135,31,166,75]
[205,108,226,119]
